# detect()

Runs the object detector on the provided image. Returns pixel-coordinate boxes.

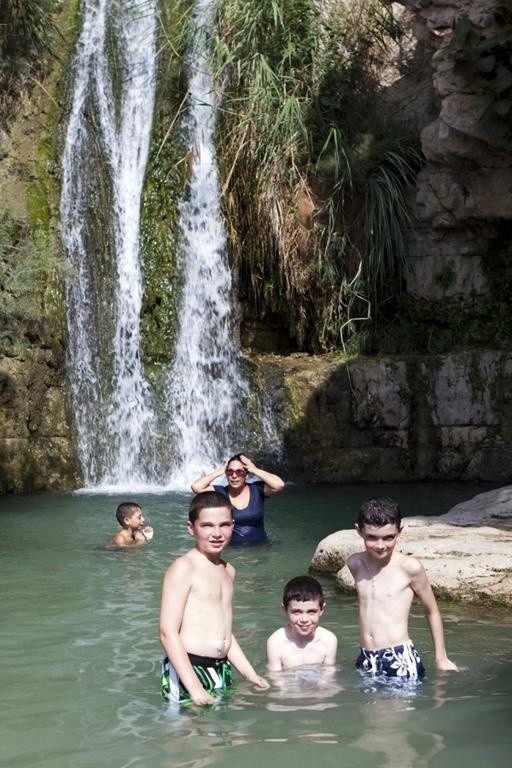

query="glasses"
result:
[224,469,249,479]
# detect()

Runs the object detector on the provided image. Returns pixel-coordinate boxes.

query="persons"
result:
[157,491,272,705]
[104,502,156,554]
[191,452,285,547]
[346,495,465,675]
[264,574,338,674]
